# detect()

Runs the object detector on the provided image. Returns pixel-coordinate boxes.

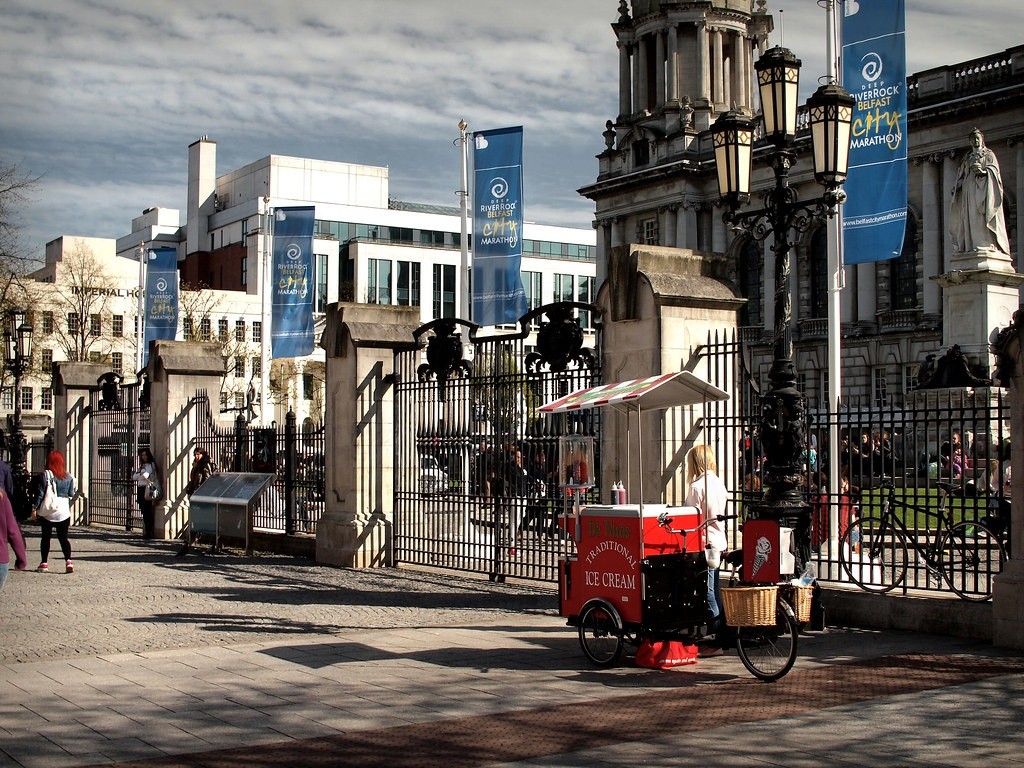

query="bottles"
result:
[800,562,817,587]
[611,480,626,505]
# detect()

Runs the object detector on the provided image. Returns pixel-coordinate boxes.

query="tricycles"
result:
[577,511,800,681]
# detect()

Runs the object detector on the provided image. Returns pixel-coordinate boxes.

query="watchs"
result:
[33,506,37,509]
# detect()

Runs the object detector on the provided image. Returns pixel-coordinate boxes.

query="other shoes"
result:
[696,646,723,658]
[506,548,522,558]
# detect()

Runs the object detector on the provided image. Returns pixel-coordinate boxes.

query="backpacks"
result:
[210,462,217,473]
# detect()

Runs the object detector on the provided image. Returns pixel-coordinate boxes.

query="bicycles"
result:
[838,468,1006,602]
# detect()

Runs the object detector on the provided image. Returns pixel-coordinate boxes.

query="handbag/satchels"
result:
[145,476,163,500]
[36,469,59,516]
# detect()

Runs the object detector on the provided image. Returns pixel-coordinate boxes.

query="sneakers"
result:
[38,562,48,573]
[65,559,75,573]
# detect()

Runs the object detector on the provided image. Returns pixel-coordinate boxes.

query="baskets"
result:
[790,586,814,622]
[719,586,778,626]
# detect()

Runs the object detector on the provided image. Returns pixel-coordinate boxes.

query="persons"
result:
[949,128,1008,254]
[684,444,729,658]
[477,430,1014,557]
[31,450,75,573]
[0,460,27,598]
[186,447,212,500]
[132,448,157,539]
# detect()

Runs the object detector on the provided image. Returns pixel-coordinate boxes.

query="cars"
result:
[420,453,448,498]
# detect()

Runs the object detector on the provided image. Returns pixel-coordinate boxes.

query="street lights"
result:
[708,45,857,631]
[3,307,33,520]
